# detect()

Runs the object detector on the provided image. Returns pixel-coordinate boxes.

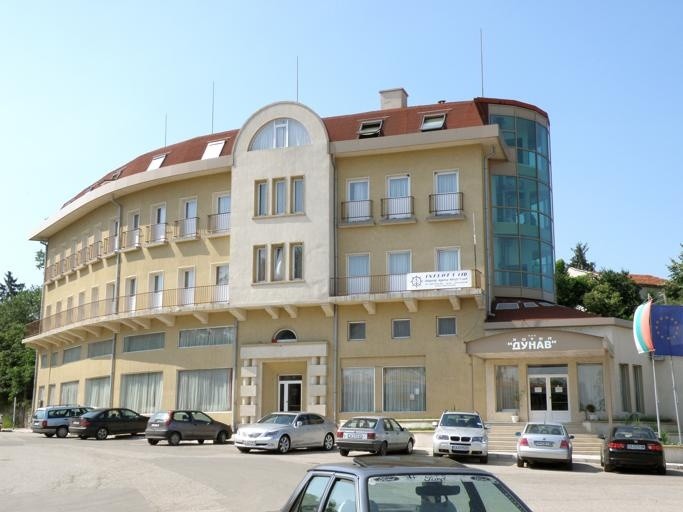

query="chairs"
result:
[418,502,457,511]
[448,418,457,425]
[111,414,119,420]
[336,497,379,512]
[465,418,477,427]
[182,415,188,419]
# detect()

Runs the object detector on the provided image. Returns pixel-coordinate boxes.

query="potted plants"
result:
[512,411,520,423]
[586,404,598,421]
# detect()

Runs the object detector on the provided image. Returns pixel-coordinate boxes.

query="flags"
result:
[650,304,683,357]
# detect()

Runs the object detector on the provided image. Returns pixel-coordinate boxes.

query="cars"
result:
[234,412,337,454]
[281,457,530,512]
[514,422,575,469]
[598,425,666,473]
[145,410,232,446]
[337,416,415,456]
[69,408,149,440]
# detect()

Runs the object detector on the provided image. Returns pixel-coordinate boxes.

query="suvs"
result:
[30,404,95,437]
[432,409,489,463]
